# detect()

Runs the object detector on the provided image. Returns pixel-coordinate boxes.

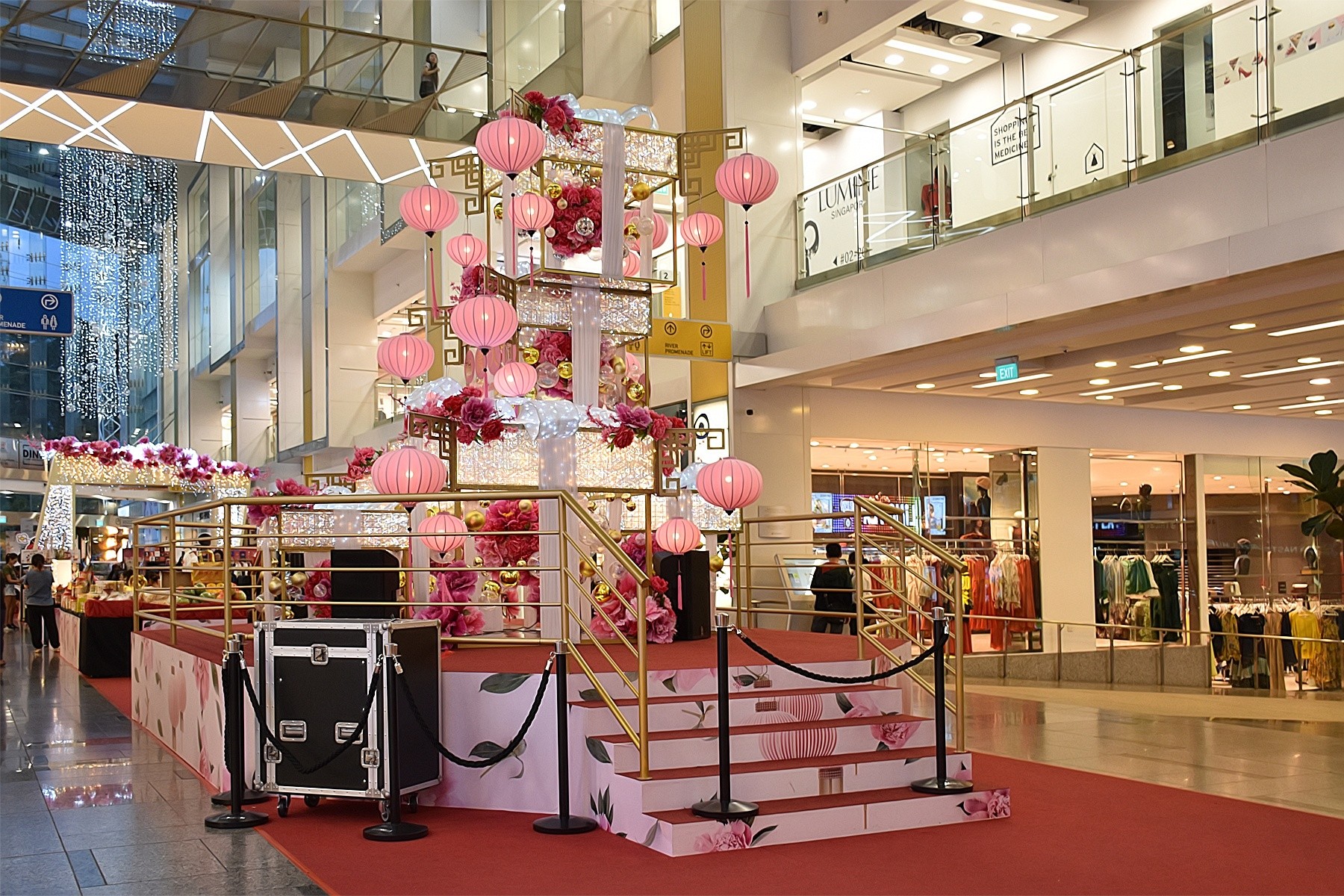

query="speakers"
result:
[330,549,401,620]
[662,551,712,641]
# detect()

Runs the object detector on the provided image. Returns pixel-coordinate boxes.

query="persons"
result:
[970,519,985,535]
[812,499,826,526]
[419,52,440,98]
[1234,538,1251,575]
[3,552,60,654]
[921,164,952,230]
[970,485,990,517]
[0,569,6,665]
[1137,483,1152,520]
[810,542,853,634]
[848,552,874,635]
[26,652,60,744]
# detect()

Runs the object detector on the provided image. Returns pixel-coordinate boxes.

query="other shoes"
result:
[0,659,6,666]
[34,648,42,653]
[3,624,18,630]
[53,647,60,651]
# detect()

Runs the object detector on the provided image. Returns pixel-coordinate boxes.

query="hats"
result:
[1237,538,1252,551]
[976,477,991,490]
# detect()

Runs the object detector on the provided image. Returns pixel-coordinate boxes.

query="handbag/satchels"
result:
[191,550,224,584]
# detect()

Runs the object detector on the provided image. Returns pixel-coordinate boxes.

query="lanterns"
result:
[697,457,763,598]
[371,445,447,618]
[399,116,554,399]
[377,332,435,386]
[623,252,640,277]
[715,153,779,298]
[623,210,668,255]
[656,516,701,610]
[493,362,537,398]
[680,210,723,300]
[418,512,468,558]
